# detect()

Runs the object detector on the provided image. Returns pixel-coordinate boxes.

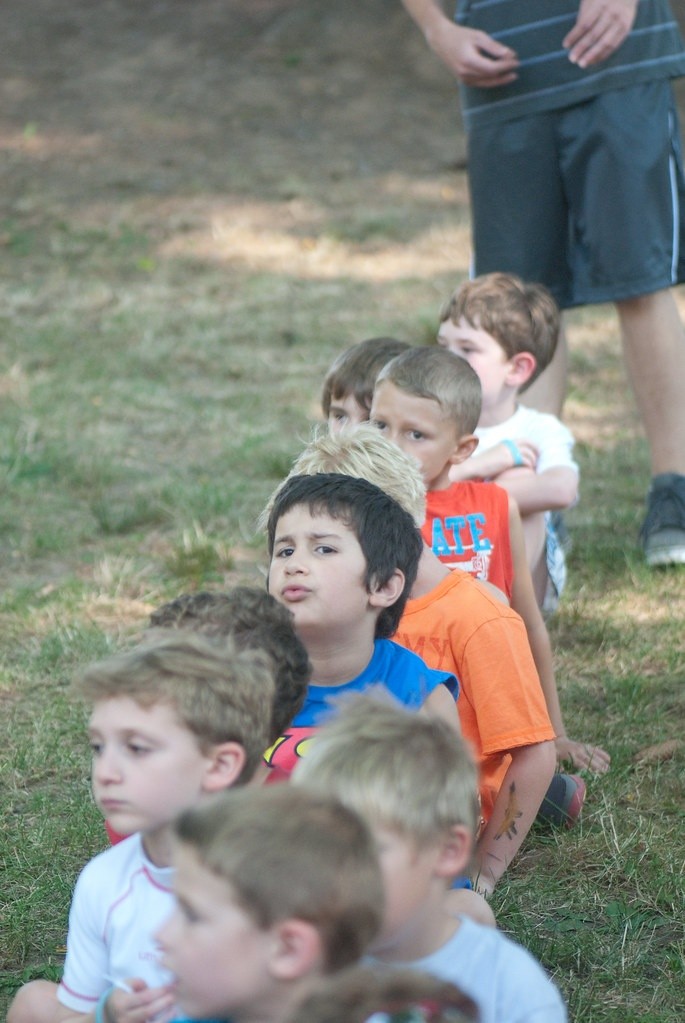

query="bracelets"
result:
[501,439,522,467]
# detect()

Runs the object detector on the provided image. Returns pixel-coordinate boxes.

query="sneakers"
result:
[636,472,685,565]
[536,773,586,829]
[553,519,574,556]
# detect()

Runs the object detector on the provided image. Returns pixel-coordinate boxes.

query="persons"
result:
[404,0,684,570]
[0,273,619,1023]
